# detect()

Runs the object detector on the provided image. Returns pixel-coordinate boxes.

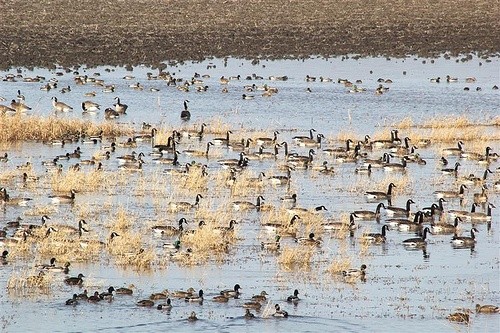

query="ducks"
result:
[286,289,300,302]
[136,288,204,309]
[447,313,470,322]
[0,66,498,121]
[475,304,499,314]
[211,284,242,303]
[251,291,269,301]
[342,264,367,278]
[272,304,288,318]
[0,127,499,264]
[25,258,136,305]
[244,309,255,318]
[242,295,261,309]
